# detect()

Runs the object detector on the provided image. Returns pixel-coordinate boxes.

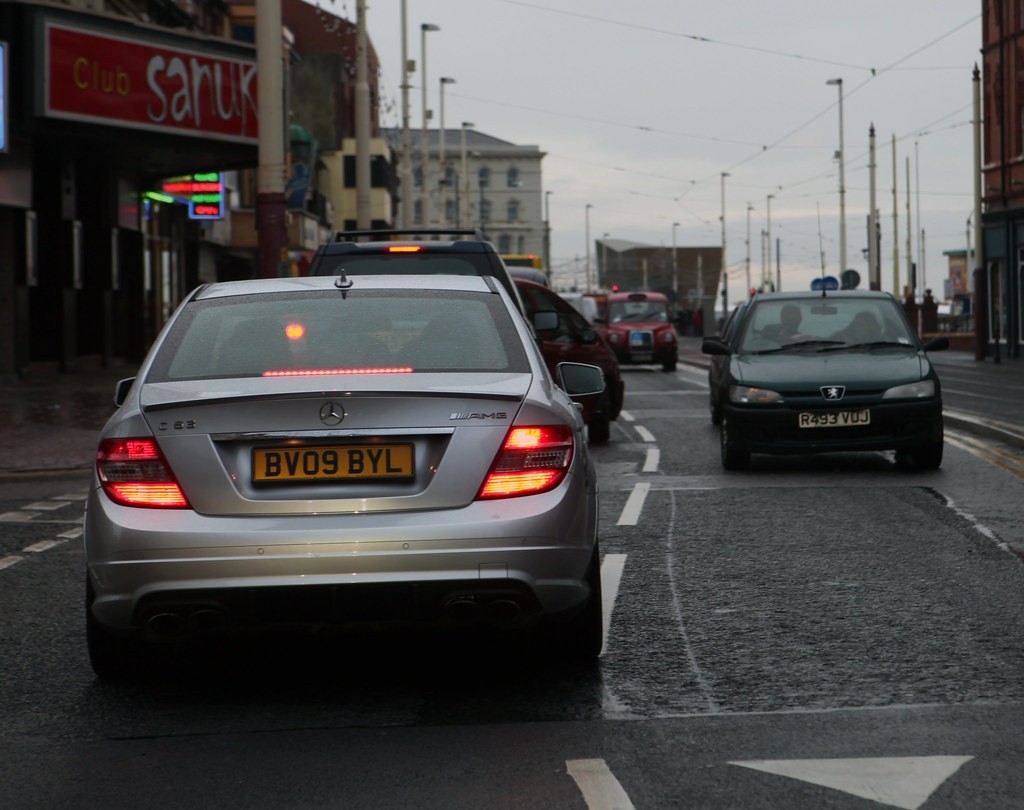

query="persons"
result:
[607,282,902,354]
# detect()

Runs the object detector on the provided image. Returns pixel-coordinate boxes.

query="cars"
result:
[312,223,534,342]
[513,277,625,444]
[701,285,944,472]
[592,288,678,372]
[82,262,605,672]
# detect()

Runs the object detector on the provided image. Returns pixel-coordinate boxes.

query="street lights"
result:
[411,17,920,323]
